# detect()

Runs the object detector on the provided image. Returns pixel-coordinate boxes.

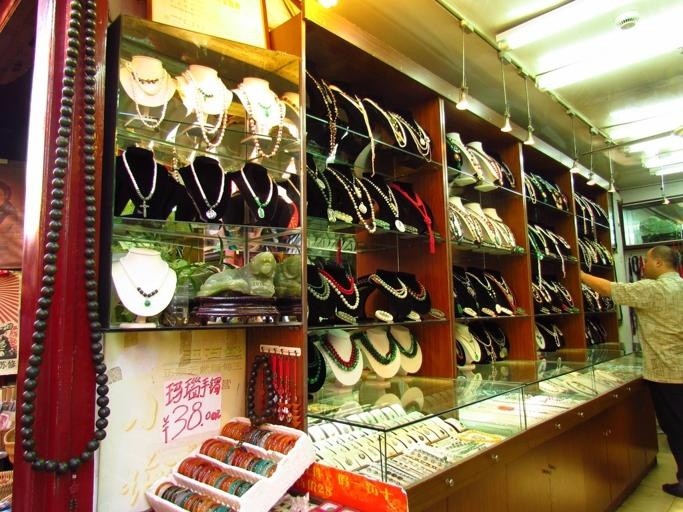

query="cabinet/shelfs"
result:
[13,0,660,511]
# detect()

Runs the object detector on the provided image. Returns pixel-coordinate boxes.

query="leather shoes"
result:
[662,483,683,498]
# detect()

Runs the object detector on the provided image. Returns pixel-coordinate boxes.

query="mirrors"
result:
[617,195,682,251]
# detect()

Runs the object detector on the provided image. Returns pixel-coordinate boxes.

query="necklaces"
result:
[368,274,426,301]
[304,60,433,386]
[521,170,613,353]
[19,1,110,479]
[120,257,170,306]
[306,267,359,310]
[305,70,433,177]
[246,348,302,428]
[307,325,417,383]
[446,125,528,367]
[306,158,432,253]
[123,151,299,220]
[126,60,300,158]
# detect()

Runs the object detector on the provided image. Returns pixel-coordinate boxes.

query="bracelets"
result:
[152,421,298,512]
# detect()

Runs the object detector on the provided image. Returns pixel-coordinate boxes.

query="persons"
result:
[580,245,683,498]
